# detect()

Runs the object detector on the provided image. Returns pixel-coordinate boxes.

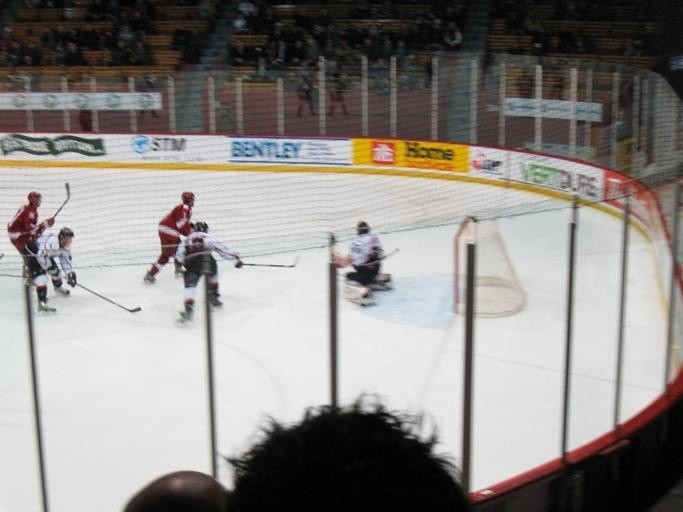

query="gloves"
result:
[46,266,58,276]
[45,218,54,226]
[67,272,76,287]
[235,261,242,268]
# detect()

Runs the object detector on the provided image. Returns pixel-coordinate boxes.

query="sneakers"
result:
[144,273,155,282]
[54,286,70,295]
[38,306,56,312]
[175,270,185,274]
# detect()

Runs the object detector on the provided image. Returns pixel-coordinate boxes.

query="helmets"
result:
[28,192,41,200]
[182,192,194,207]
[357,222,368,235]
[196,222,208,232]
[58,228,74,240]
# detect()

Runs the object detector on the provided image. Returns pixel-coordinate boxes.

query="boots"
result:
[180,304,194,319]
[209,293,222,306]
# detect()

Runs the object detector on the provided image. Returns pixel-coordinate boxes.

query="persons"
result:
[173,219,242,317]
[20,227,77,311]
[121,471,232,512]
[146,188,194,280]
[230,390,476,512]
[332,219,392,305]
[7,192,54,285]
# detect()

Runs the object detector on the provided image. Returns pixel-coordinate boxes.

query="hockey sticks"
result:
[242,256,300,267]
[64,276,141,312]
[53,183,69,218]
